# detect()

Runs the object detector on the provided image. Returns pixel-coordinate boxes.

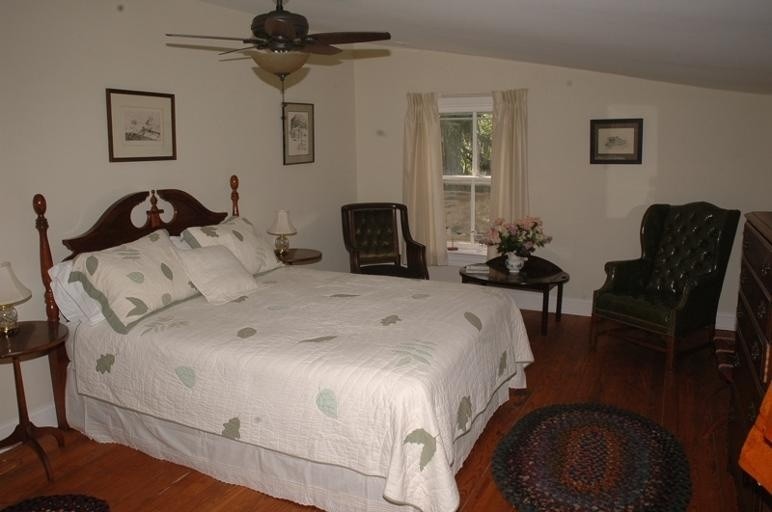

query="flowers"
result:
[470,208,553,257]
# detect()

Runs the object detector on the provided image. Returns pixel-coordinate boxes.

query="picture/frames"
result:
[104,86,178,165]
[589,116,644,164]
[281,98,316,166]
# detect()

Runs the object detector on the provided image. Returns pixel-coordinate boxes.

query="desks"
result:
[457,261,572,338]
[1,318,71,488]
[273,247,322,266]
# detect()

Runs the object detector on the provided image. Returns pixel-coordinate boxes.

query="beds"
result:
[32,173,534,512]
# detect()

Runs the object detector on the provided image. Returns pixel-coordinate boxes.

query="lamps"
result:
[243,48,309,82]
[267,210,299,256]
[1,261,34,337]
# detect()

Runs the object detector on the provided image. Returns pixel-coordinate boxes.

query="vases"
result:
[504,255,524,273]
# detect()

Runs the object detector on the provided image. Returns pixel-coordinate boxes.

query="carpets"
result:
[487,398,693,510]
[2,491,107,512]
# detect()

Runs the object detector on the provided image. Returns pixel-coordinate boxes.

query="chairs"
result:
[336,196,429,281]
[584,200,747,376]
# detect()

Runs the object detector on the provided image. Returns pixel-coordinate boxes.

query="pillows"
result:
[178,212,285,279]
[67,224,202,335]
[166,243,262,307]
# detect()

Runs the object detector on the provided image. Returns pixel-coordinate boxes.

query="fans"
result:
[166,1,392,58]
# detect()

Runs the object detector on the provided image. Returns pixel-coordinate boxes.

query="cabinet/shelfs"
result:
[729,205,772,512]
